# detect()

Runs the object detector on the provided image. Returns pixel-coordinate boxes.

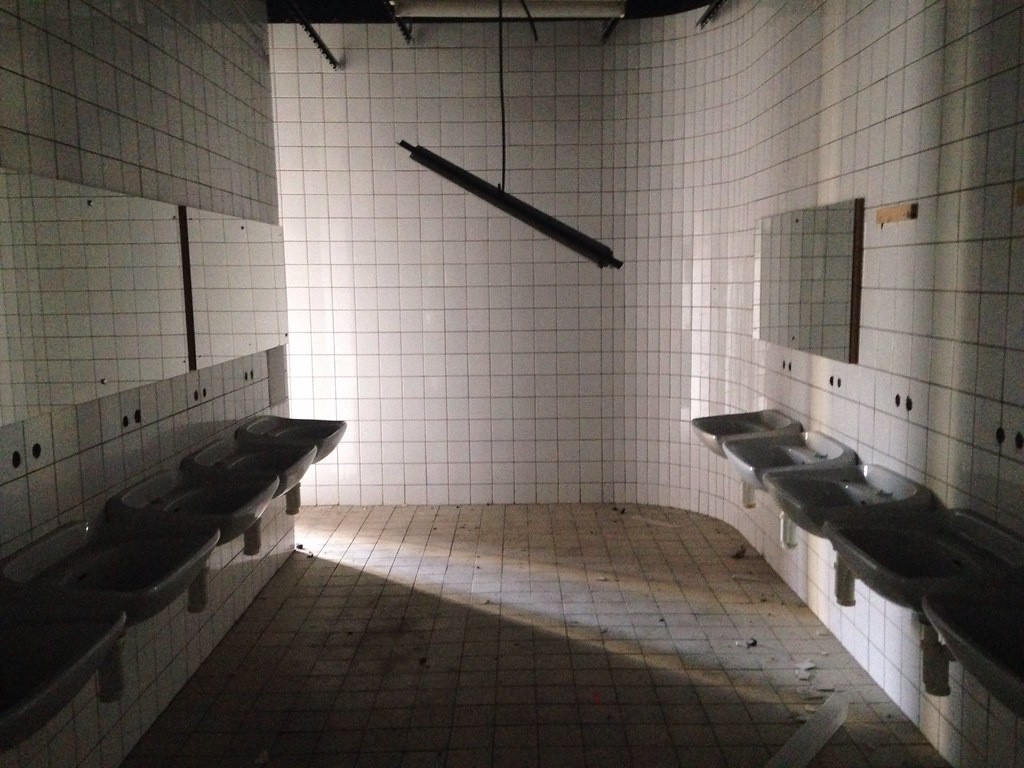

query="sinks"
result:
[722,426,859,491]
[919,586,1024,717]
[0,608,128,754]
[29,528,222,626]
[237,413,345,463]
[104,466,281,548]
[181,434,318,498]
[690,405,803,459]
[762,461,935,541]
[822,507,1024,614]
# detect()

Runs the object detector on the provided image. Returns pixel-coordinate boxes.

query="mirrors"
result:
[752,197,864,364]
